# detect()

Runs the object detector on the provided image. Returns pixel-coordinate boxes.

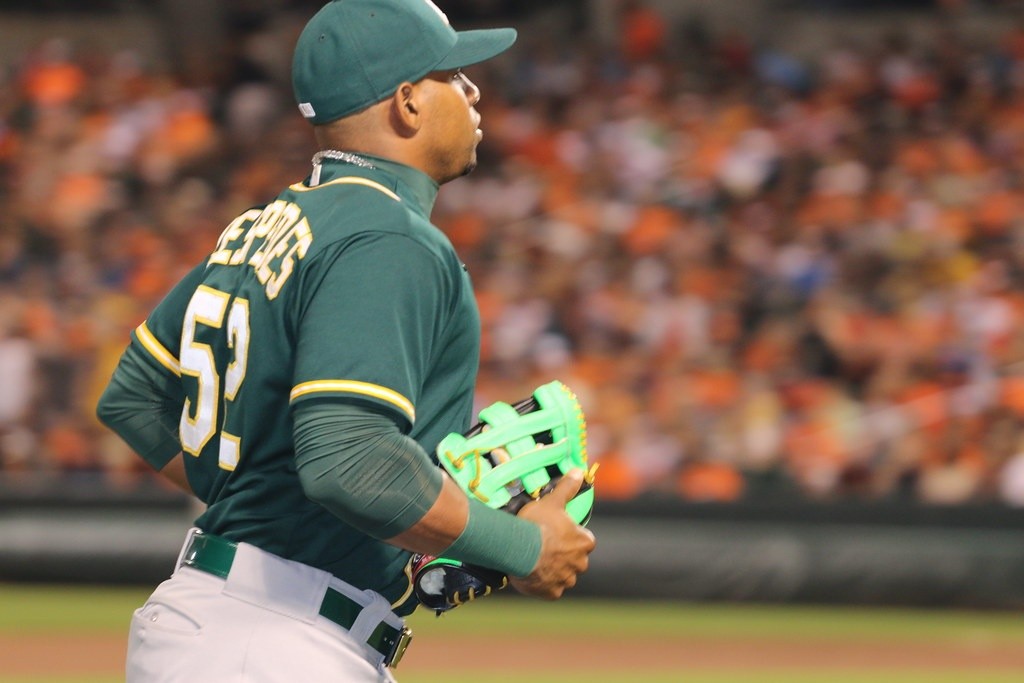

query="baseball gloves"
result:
[414,380,596,610]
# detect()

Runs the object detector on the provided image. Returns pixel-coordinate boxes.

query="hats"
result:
[291,0,517,126]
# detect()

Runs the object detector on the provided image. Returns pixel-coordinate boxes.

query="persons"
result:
[0,0,1023,511]
[96,1,594,683]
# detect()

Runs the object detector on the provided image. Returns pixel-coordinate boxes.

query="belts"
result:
[181,532,413,668]
